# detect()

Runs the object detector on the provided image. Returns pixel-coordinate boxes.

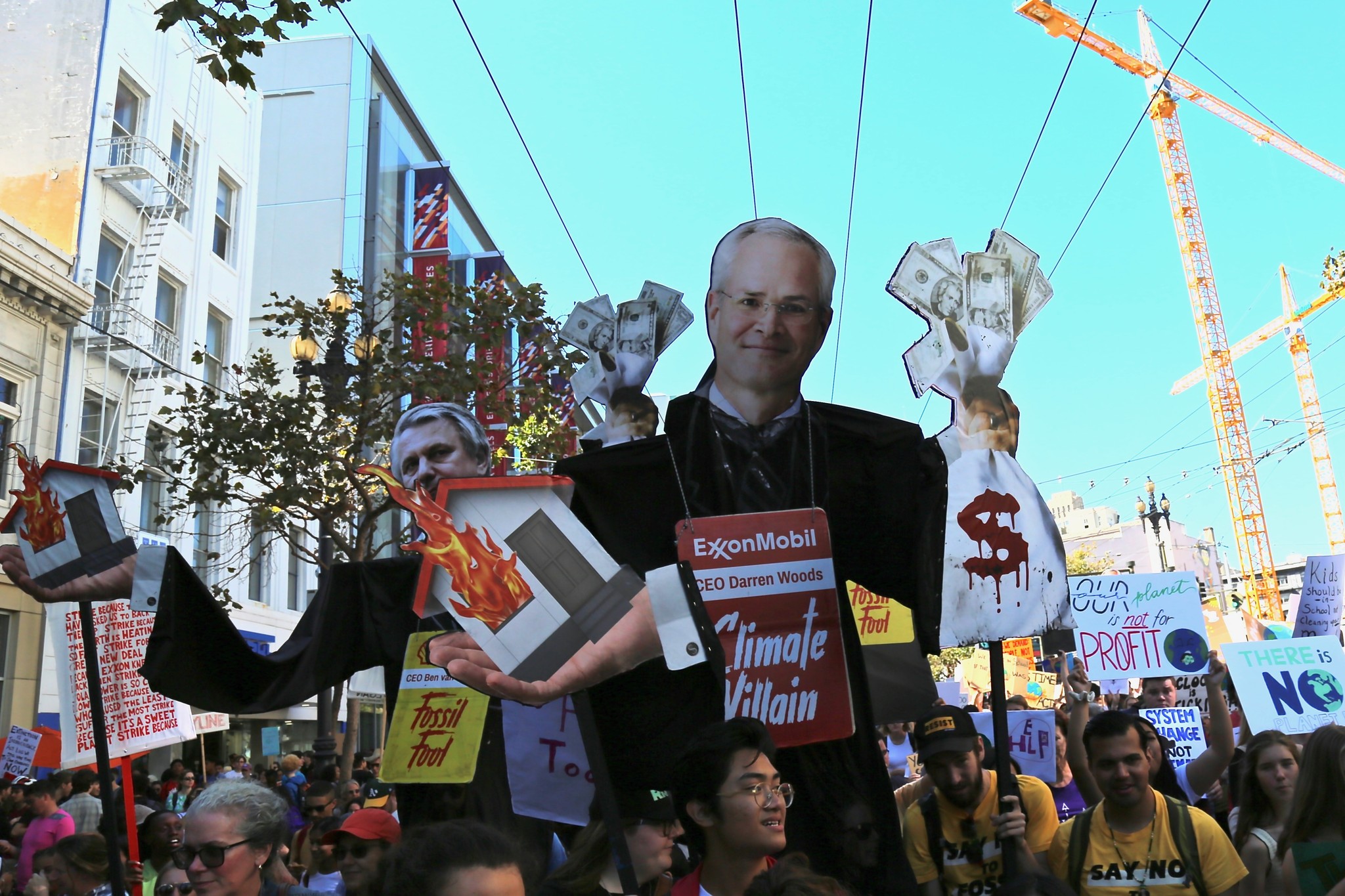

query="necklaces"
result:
[1102,798,1157,896]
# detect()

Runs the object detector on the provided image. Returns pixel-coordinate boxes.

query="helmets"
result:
[12,775,38,786]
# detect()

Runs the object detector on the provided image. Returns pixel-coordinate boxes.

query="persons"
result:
[427,559,721,710]
[667,715,795,896]
[0,750,854,896]
[0,391,659,830]
[875,649,1345,896]
[559,217,1023,896]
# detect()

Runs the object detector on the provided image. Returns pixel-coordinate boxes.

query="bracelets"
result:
[1068,690,1095,702]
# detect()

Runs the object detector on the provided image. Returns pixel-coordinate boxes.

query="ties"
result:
[707,402,806,512]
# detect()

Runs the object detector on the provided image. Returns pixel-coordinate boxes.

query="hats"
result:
[229,753,238,760]
[321,808,402,845]
[589,774,679,826]
[291,751,304,758]
[241,764,252,771]
[363,778,393,807]
[249,772,259,778]
[913,704,977,761]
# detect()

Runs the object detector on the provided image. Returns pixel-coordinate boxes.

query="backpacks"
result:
[286,779,311,807]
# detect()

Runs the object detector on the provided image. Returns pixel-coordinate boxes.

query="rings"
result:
[989,412,999,430]
[631,410,637,422]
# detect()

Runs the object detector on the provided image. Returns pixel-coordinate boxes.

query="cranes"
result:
[1169,261,1344,557]
[1013,1,1344,624]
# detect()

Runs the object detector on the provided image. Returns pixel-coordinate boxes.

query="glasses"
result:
[718,289,822,325]
[370,763,379,768]
[349,789,359,794]
[331,844,379,860]
[170,838,257,870]
[154,788,160,791]
[11,790,22,794]
[274,766,278,769]
[713,782,796,810]
[302,798,334,815]
[634,813,682,837]
[156,882,193,896]
[182,776,196,780]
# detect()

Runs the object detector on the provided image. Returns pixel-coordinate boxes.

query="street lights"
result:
[289,275,386,783]
[1135,473,1177,573]
